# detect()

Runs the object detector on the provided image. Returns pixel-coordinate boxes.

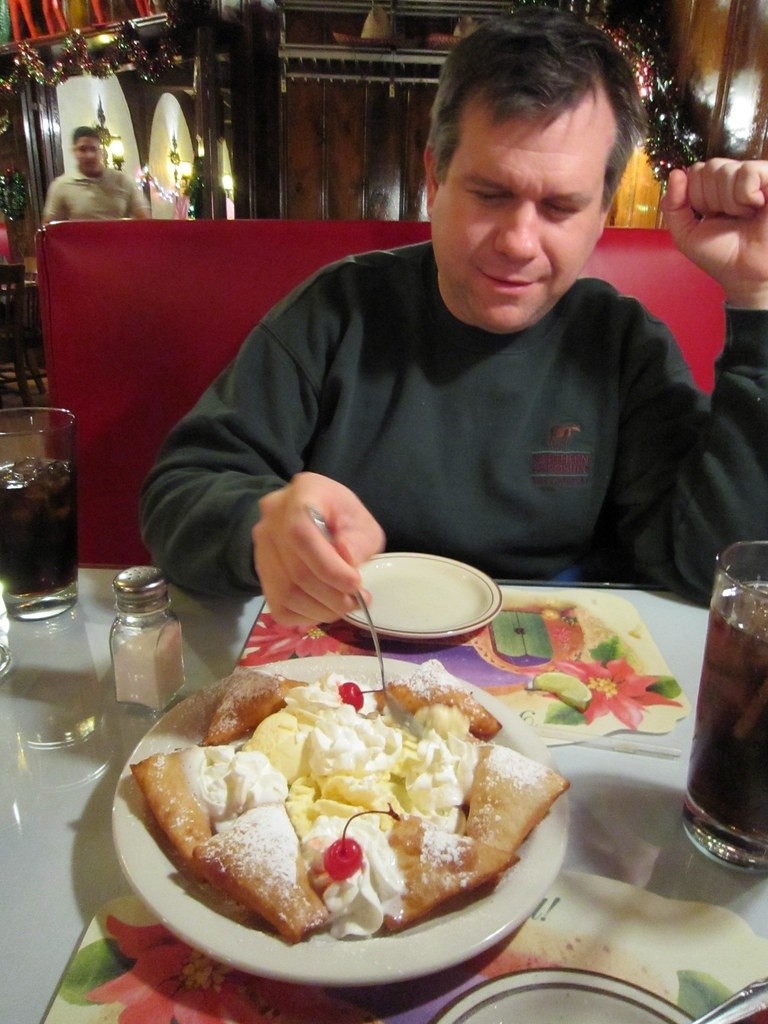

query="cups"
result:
[3,612,110,795]
[0,406,80,622]
[679,539,767,876]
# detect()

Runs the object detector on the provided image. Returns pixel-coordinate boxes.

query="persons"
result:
[43,124,148,222]
[136,6,767,632]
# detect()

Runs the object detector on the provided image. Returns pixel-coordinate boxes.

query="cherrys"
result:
[340,680,389,708]
[321,802,404,880]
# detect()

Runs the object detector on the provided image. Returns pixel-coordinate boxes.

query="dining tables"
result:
[0,570,766,1024]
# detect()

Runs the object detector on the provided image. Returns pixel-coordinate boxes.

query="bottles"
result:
[110,563,189,716]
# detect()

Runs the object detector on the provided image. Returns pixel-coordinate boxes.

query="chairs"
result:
[1,264,33,414]
[35,215,732,569]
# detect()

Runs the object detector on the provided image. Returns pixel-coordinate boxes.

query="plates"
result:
[428,967,696,1023]
[112,656,574,983]
[335,549,505,645]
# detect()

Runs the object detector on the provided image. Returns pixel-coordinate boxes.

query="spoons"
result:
[302,500,426,742]
[693,973,767,1024]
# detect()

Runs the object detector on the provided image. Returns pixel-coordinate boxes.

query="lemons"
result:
[533,670,593,709]
